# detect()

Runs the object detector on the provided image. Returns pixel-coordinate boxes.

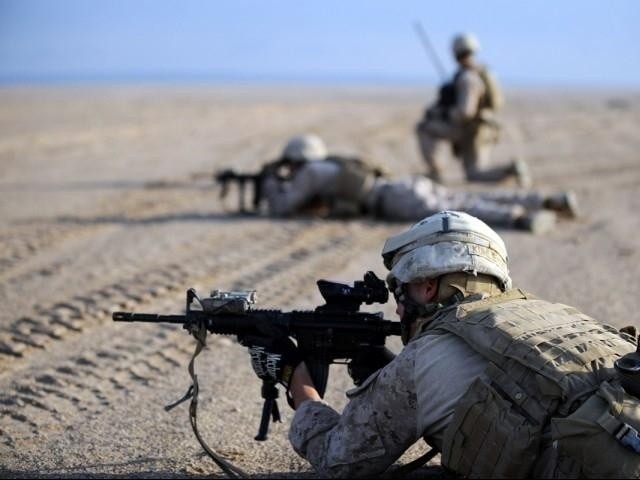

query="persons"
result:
[287,209,639,480]
[416,32,531,188]
[259,132,583,237]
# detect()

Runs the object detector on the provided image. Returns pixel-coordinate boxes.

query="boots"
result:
[505,158,532,189]
[514,191,579,237]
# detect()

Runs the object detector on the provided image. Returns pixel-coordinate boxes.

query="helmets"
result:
[285,132,329,165]
[382,211,510,291]
[452,33,479,59]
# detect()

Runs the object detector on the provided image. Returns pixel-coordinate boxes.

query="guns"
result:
[113,272,405,441]
[192,159,295,208]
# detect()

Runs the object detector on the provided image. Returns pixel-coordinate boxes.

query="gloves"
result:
[249,333,305,394]
